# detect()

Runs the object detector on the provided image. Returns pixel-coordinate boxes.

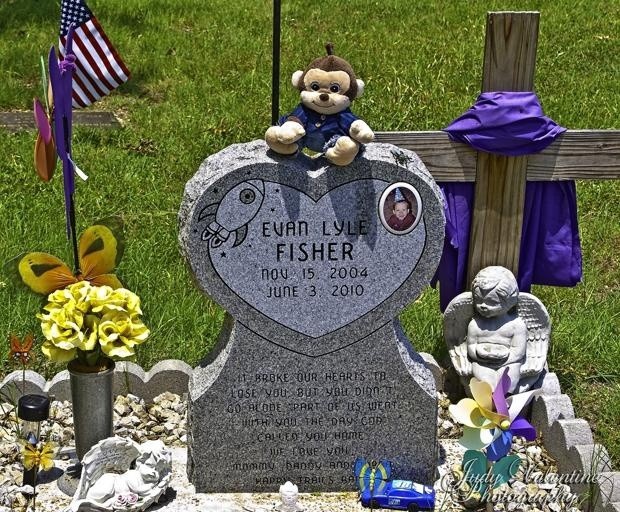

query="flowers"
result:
[34,277,152,366]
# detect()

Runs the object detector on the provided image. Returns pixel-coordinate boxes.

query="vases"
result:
[67,358,115,462]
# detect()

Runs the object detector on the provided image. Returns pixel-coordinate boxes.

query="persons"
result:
[387,201,416,229]
[265,44,374,167]
[72,438,173,512]
[440,266,553,418]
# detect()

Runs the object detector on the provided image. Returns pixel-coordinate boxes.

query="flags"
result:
[58,0,132,108]
